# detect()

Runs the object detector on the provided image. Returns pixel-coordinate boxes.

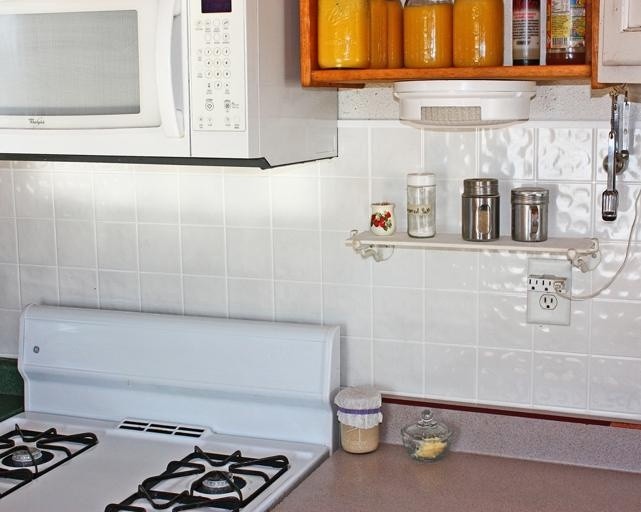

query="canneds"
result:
[333,386,384,454]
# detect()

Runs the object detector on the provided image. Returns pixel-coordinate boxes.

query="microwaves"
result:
[0,0,340,170]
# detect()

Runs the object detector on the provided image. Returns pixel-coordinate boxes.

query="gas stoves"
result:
[0,302,346,511]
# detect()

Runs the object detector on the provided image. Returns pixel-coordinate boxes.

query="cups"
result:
[509,186,551,240]
[370,201,395,238]
[406,173,438,240]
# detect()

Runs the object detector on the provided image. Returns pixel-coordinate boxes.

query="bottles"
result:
[459,179,502,241]
[319,1,585,66]
[336,382,382,453]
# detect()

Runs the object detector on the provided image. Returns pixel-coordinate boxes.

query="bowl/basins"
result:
[401,408,453,462]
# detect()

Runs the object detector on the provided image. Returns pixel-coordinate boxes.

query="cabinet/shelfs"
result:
[300,0,609,90]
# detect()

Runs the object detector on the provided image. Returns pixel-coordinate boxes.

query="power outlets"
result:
[528,276,571,326]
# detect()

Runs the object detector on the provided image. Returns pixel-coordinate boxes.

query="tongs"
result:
[602,131,618,221]
